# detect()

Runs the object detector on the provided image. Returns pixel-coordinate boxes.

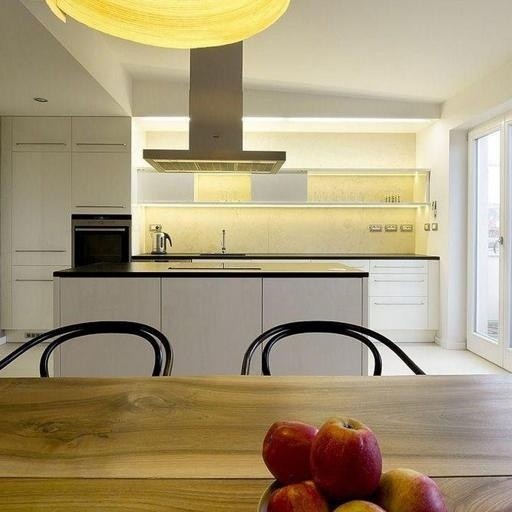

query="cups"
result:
[314,191,364,202]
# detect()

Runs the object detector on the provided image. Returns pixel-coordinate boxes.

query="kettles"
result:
[149,226,172,253]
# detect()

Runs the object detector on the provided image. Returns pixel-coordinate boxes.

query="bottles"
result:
[384,193,400,202]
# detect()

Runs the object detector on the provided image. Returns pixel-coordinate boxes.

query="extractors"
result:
[142,40,286,174]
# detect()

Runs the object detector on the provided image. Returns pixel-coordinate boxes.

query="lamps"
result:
[45,0,292,48]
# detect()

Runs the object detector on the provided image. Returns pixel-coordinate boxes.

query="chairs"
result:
[0,321,175,377]
[239,320,427,374]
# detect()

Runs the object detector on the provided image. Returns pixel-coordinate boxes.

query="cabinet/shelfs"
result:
[0,150,138,266]
[0,115,146,153]
[0,263,67,343]
[362,258,442,343]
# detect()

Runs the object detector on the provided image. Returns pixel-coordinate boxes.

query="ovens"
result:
[70,214,131,271]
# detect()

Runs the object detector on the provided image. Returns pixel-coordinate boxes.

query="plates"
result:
[257,478,278,511]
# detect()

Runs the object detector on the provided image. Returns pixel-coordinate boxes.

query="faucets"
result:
[221,229,226,252]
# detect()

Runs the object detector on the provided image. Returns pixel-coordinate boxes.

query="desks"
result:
[51,262,372,379]
[1,374,511,512]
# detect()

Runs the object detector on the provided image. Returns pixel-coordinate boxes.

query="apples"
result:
[332,499,385,512]
[261,420,318,485]
[379,468,447,512]
[267,480,330,512]
[311,416,382,500]
